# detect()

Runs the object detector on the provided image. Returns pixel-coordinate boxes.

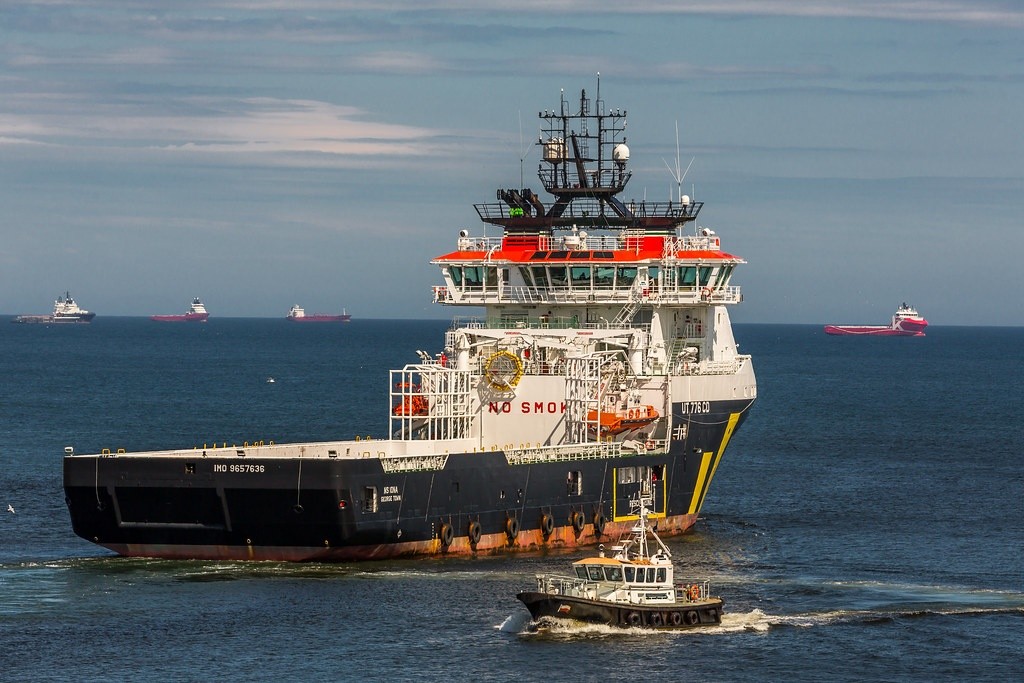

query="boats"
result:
[823,301,929,337]
[515,466,725,629]
[285,304,352,321]
[149,296,210,323]
[62,71,758,562]
[15,289,96,324]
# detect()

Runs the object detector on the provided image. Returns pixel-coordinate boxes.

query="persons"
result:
[547,311,556,329]
[652,465,662,481]
[578,272,628,290]
[571,315,579,329]
[686,581,702,602]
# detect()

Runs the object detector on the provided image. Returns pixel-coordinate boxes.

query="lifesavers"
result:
[573,511,586,533]
[628,409,634,419]
[468,521,481,544]
[542,514,554,535]
[626,610,699,627]
[645,441,655,450]
[684,362,688,370]
[440,522,453,547]
[635,409,640,418]
[593,511,605,534]
[689,585,699,600]
[702,287,710,297]
[506,517,519,539]
